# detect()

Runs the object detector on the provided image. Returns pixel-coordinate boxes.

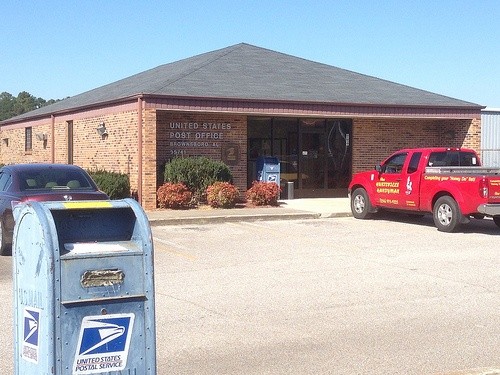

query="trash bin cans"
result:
[10,197,159,375]
[256,156,281,200]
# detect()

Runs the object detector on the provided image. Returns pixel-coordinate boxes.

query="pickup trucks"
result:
[348,146,500,233]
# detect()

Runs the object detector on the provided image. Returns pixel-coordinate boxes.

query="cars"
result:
[1,163,110,254]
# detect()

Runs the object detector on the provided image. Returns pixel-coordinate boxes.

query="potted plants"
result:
[156,183,195,209]
[206,181,239,208]
[246,179,282,207]
